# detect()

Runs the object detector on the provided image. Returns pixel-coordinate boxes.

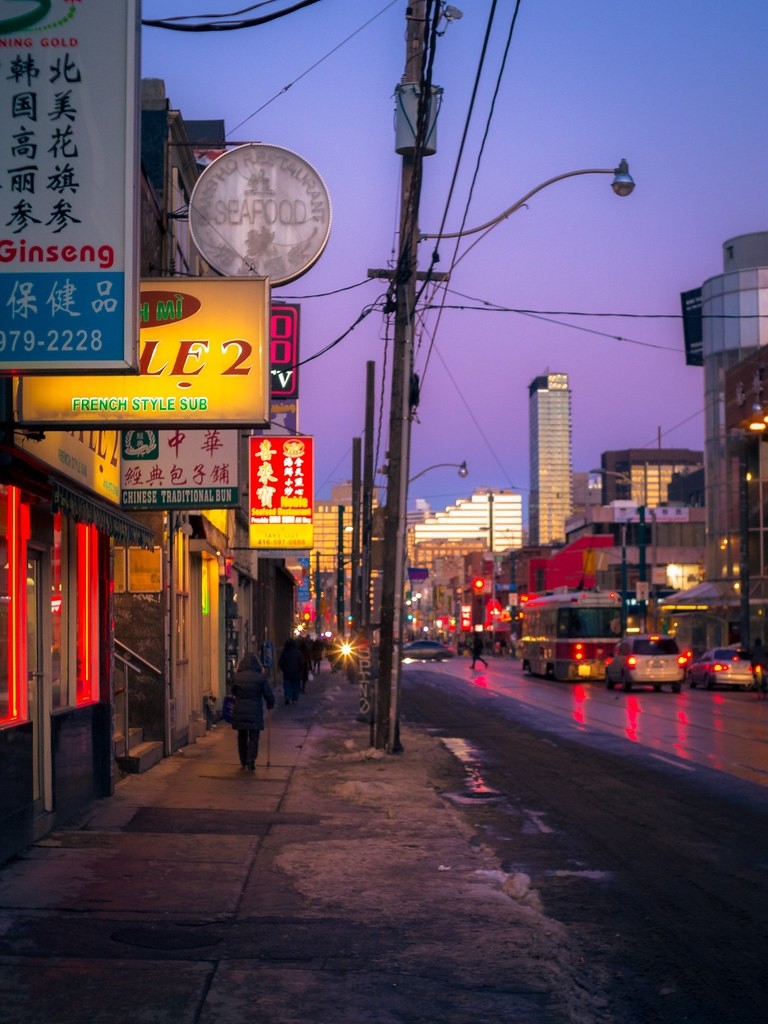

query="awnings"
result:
[663,580,737,599]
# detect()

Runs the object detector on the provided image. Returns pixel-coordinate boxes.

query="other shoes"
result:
[287,699,292,704]
[292,699,297,704]
[248,762,255,770]
[242,761,246,767]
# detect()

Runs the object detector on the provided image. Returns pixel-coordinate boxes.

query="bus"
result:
[516,591,627,683]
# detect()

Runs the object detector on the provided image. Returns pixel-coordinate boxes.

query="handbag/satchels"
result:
[222,694,235,722]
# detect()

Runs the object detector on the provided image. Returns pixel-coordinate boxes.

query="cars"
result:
[605,633,686,693]
[689,646,763,690]
[399,638,457,662]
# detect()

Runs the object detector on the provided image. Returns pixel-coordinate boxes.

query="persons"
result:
[749,638,768,700]
[278,638,305,706]
[501,637,507,656]
[469,633,488,669]
[297,634,324,674]
[230,652,275,770]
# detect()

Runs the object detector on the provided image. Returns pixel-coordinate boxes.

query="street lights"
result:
[588,466,649,633]
[351,461,470,682]
[479,526,518,658]
[372,157,635,755]
